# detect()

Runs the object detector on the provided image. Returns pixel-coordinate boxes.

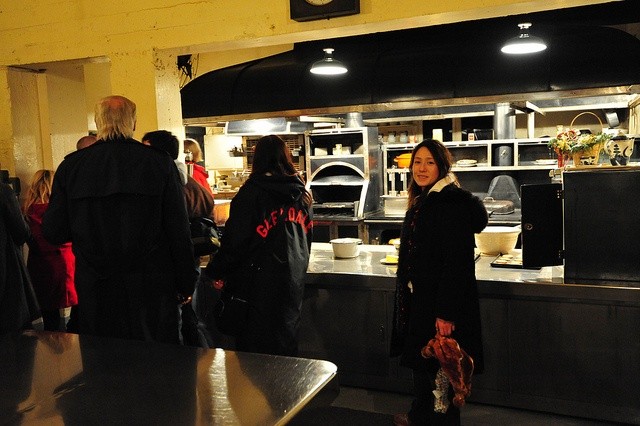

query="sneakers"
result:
[395,409,407,425]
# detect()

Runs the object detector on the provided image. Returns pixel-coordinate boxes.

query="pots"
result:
[331,236,362,256]
[393,153,418,169]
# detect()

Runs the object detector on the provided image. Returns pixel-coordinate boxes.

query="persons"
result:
[389,139,488,426]
[21,169,79,323]
[40,95,196,341]
[197,135,314,357]
[0,178,42,337]
[141,130,215,223]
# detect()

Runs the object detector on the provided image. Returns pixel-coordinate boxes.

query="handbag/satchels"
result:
[190,217,222,256]
[192,272,247,336]
[399,329,483,412]
[195,187,302,336]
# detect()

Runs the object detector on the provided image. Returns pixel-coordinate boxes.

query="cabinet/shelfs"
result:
[376,84,640,223]
[519,170,640,284]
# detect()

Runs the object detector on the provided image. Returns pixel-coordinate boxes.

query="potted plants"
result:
[568,135,603,165]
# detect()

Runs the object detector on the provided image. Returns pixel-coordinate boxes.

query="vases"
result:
[549,139,566,167]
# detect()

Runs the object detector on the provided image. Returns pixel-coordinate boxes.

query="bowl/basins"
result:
[477,225,516,257]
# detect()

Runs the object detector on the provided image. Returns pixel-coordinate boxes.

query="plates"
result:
[457,158,477,165]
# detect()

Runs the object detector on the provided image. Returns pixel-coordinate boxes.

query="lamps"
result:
[308,48,348,77]
[500,23,548,56]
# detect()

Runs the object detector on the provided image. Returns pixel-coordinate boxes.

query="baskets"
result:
[570,112,604,165]
